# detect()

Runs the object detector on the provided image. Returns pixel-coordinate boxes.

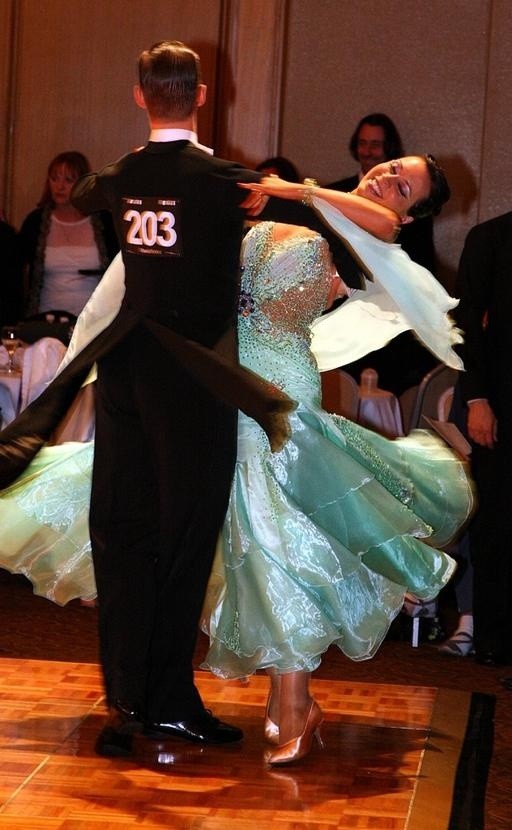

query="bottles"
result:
[360,368,378,394]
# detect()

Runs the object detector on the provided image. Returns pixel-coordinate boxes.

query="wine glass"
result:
[2,327,21,375]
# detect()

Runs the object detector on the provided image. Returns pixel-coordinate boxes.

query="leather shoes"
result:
[437,632,512,687]
[96,698,244,756]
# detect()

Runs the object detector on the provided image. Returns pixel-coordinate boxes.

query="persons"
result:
[0,153,481,769]
[2,110,512,687]
[1,40,377,747]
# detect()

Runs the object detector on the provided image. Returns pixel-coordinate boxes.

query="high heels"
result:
[264,683,327,763]
